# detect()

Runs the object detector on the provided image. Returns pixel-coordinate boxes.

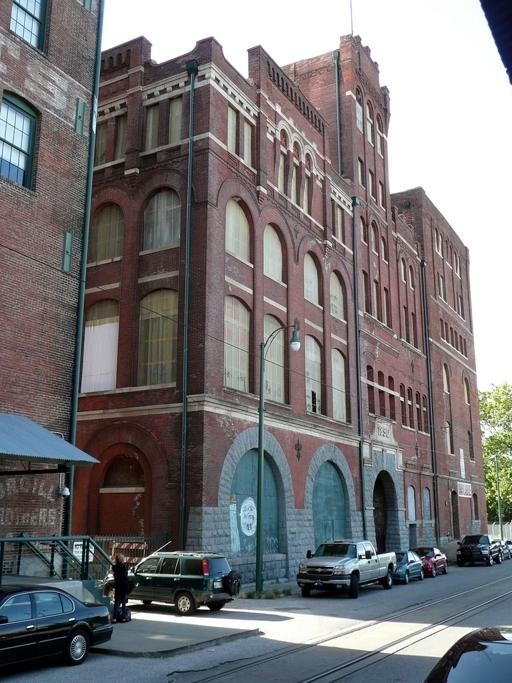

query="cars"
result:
[1,583,112,673]
[298,533,447,602]
[423,625,512,683]
[453,531,512,566]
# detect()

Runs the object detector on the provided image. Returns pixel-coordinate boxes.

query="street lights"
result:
[490,445,512,538]
[252,315,303,602]
[132,493,147,541]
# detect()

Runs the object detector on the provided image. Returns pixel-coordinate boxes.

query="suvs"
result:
[103,549,242,617]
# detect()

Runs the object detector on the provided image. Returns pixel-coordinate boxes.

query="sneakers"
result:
[112,618,127,623]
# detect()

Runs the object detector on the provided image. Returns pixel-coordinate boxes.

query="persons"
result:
[113,554,136,622]
